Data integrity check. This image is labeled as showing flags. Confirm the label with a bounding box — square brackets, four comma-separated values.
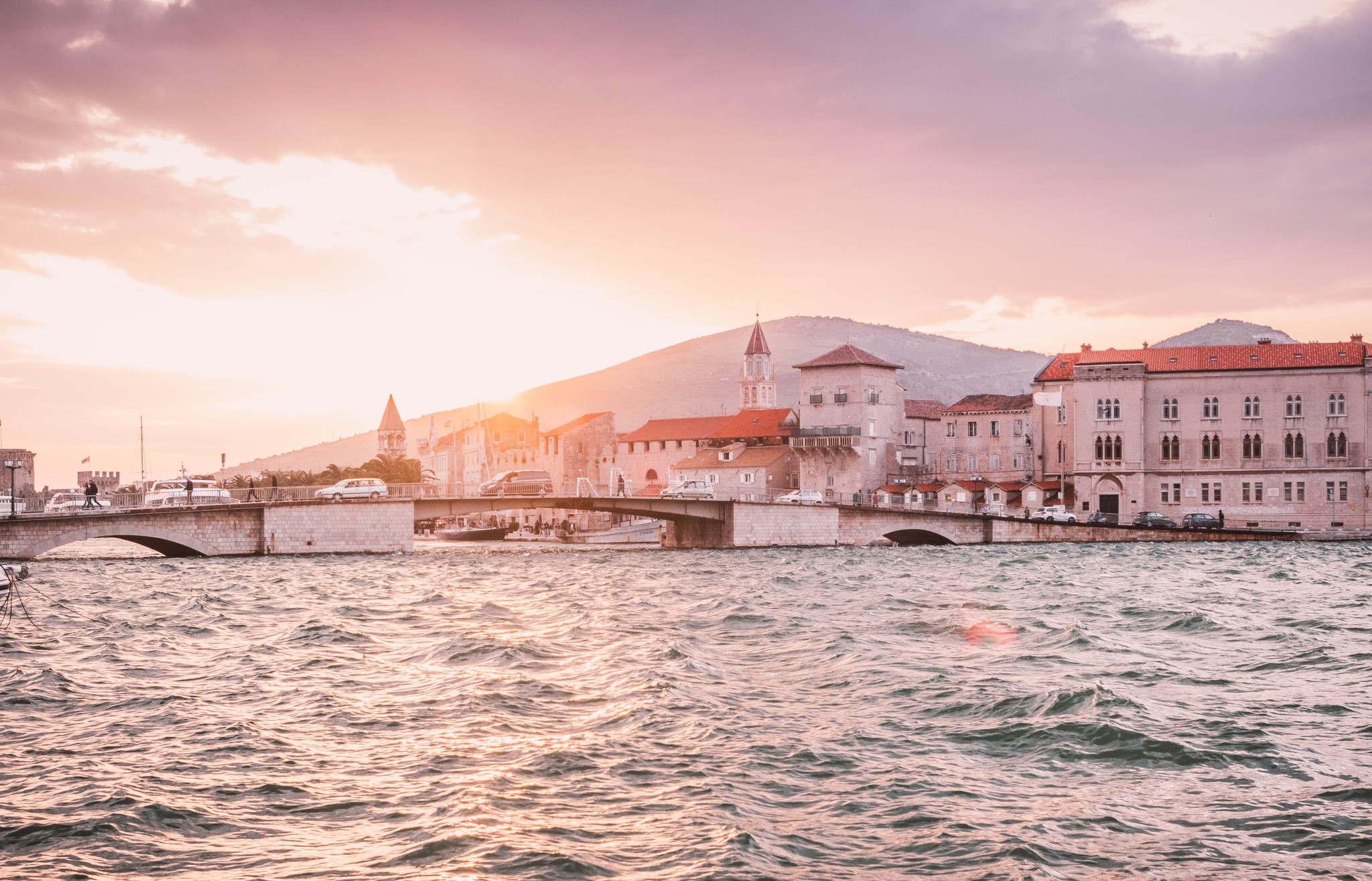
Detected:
[82, 456, 90, 464]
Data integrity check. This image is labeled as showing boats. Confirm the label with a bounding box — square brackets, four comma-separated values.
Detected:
[554, 512, 667, 543]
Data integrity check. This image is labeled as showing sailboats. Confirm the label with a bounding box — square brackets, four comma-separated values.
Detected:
[432, 401, 514, 542]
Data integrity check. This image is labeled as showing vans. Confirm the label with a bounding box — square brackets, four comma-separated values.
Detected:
[478, 470, 553, 498]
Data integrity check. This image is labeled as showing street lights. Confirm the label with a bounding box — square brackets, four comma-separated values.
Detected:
[3, 459, 23, 516]
[970, 474, 982, 513]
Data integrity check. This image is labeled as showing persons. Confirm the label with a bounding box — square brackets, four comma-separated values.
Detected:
[873, 492, 878, 507]
[267, 474, 278, 501]
[1024, 506, 1030, 518]
[570, 521, 574, 530]
[981, 505, 987, 514]
[82, 480, 103, 510]
[1218, 510, 1224, 523]
[853, 490, 864, 506]
[246, 478, 259, 502]
[1096, 509, 1102, 519]
[183, 478, 194, 505]
[539, 521, 549, 538]
[617, 475, 625, 496]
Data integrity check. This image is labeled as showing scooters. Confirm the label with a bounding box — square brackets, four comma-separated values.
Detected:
[1086, 513, 1107, 524]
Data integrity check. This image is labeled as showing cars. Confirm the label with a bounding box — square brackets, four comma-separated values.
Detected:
[1031, 507, 1078, 523]
[1182, 513, 1221, 529]
[314, 478, 389, 501]
[1132, 512, 1177, 528]
[660, 479, 714, 499]
[777, 489, 824, 504]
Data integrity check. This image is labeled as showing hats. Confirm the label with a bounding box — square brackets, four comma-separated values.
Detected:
[1219, 510, 1222, 511]
[618, 475, 622, 476]
[84, 481, 88, 484]
